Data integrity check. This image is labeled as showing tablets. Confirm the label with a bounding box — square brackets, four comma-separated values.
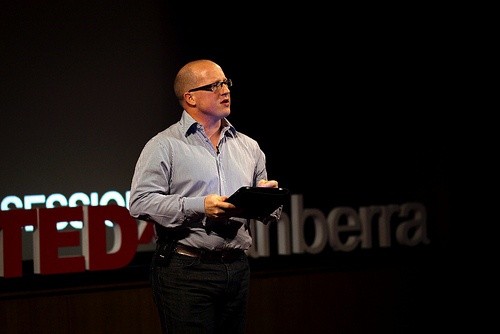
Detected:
[218, 186, 289, 220]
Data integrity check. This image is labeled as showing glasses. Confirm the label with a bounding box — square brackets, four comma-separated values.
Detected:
[188, 79, 232, 92]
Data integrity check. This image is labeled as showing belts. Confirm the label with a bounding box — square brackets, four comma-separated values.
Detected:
[159, 241, 248, 264]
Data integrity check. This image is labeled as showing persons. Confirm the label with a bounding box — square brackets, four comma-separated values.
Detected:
[130, 60, 283, 334]
